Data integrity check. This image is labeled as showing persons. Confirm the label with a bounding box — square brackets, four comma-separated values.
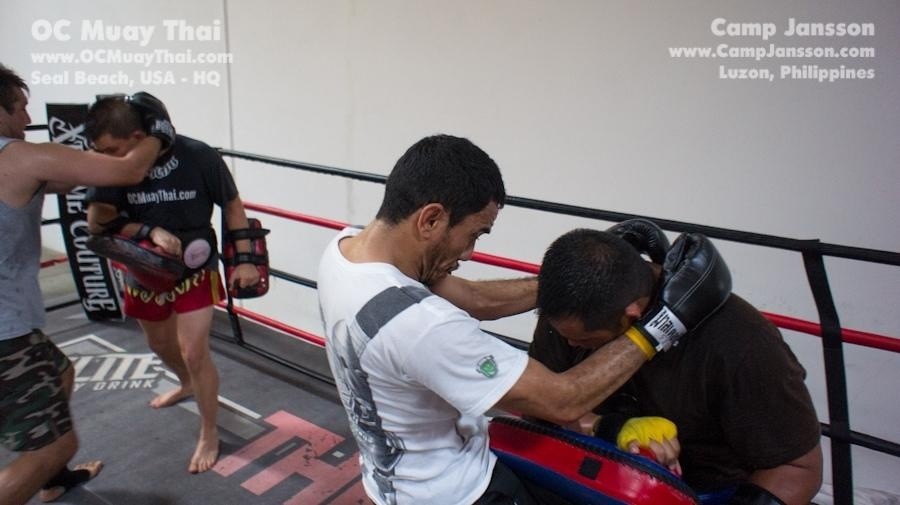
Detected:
[1, 60, 181, 505]
[525, 226, 825, 505]
[80, 92, 262, 477]
[314, 133, 734, 505]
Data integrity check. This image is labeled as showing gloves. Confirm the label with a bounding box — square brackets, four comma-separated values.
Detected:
[128, 90, 175, 157]
[604, 219, 671, 265]
[634, 229, 732, 353]
